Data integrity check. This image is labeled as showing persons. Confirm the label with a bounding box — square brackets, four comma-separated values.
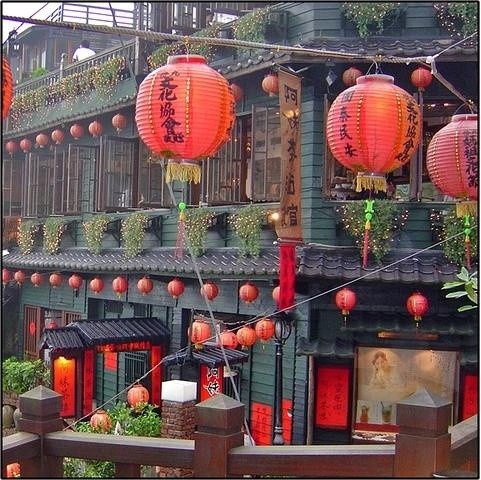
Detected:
[71, 41, 97, 63]
[370, 352, 402, 390]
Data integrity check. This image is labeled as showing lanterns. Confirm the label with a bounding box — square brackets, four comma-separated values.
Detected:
[3, 57, 14, 119]
[188, 320, 274, 351]
[343, 65, 363, 88]
[5, 112, 128, 158]
[408, 290, 428, 327]
[426, 113, 478, 272]
[201, 364, 223, 402]
[7, 463, 20, 477]
[134, 54, 236, 252]
[260, 73, 279, 97]
[1, 270, 280, 303]
[326, 73, 425, 268]
[91, 409, 110, 432]
[335, 287, 357, 323]
[127, 384, 149, 408]
[231, 83, 243, 104]
[411, 66, 433, 106]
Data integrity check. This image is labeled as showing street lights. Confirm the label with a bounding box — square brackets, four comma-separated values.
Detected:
[7, 27, 18, 81]
[267, 310, 295, 444]
